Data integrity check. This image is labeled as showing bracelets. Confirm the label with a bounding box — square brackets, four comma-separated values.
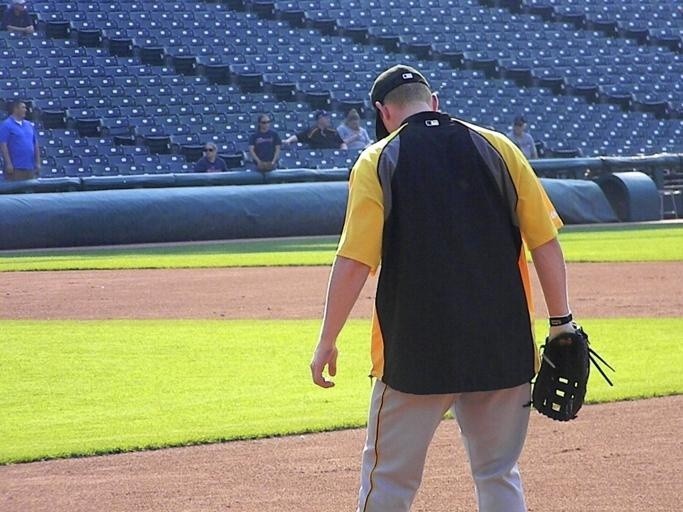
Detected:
[549, 313, 573, 326]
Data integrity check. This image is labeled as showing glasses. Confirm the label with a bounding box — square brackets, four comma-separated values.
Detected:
[259, 121, 270, 125]
[204, 149, 215, 152]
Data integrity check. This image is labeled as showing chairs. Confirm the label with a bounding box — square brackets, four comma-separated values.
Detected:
[0, 2, 683, 188]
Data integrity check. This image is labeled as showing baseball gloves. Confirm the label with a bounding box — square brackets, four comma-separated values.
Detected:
[533, 323, 589, 422]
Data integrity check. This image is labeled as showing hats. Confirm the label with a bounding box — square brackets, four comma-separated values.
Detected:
[370, 63, 432, 141]
[314, 108, 333, 120]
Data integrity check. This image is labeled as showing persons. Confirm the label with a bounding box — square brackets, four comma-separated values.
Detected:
[309, 65, 577, 512]
[192, 141, 229, 174]
[336, 109, 372, 149]
[281, 109, 349, 150]
[248, 113, 283, 171]
[2, 0, 35, 34]
[506, 116, 539, 161]
[0, 100, 42, 181]
